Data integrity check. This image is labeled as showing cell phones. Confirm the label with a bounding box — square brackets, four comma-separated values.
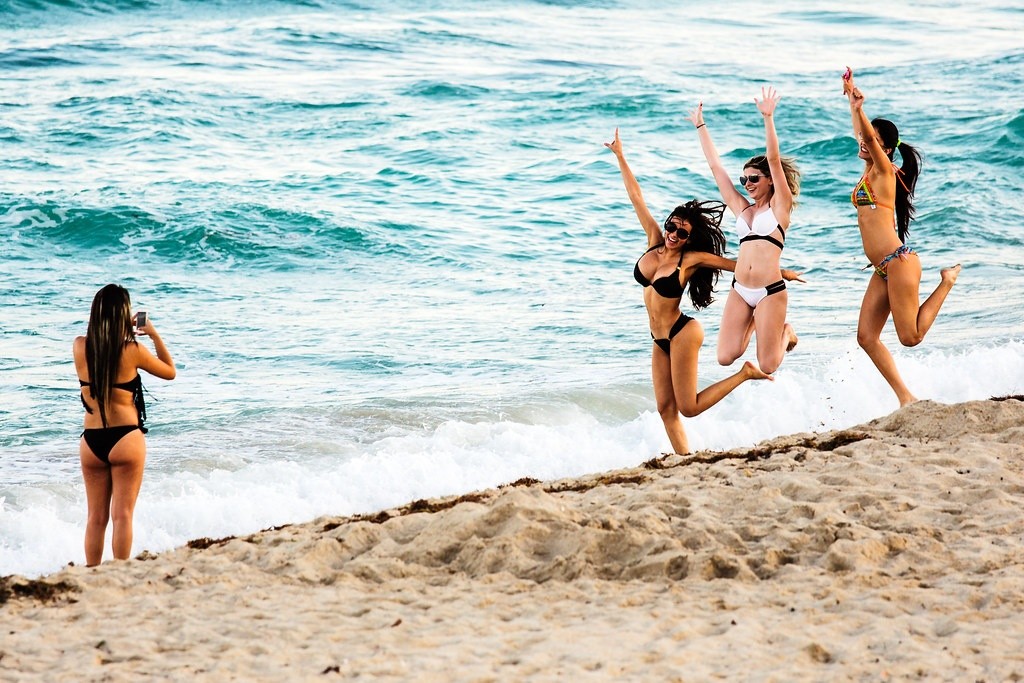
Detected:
[136, 311, 147, 330]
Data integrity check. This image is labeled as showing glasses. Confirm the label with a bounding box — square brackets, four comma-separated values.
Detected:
[740, 173, 767, 186]
[858, 129, 864, 141]
[664, 221, 690, 239]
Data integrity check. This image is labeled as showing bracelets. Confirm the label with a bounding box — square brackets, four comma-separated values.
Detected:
[697, 124, 705, 129]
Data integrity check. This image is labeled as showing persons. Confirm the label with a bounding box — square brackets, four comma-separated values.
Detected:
[603, 127, 807, 457]
[843, 66, 961, 408]
[684, 86, 801, 374]
[73, 284, 177, 566]
